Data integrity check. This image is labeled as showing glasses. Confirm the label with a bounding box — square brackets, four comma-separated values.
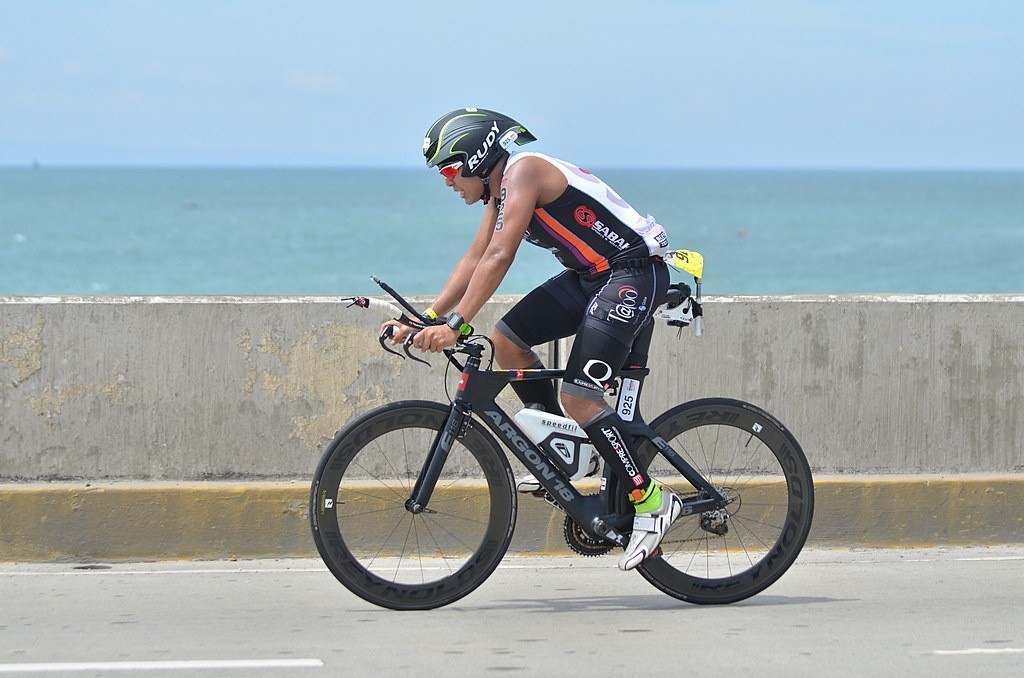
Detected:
[438, 160, 466, 179]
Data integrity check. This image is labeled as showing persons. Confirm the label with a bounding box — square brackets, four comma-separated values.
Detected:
[378, 109, 683, 571]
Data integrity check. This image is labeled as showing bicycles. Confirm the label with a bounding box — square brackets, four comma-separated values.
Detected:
[310, 275, 815, 612]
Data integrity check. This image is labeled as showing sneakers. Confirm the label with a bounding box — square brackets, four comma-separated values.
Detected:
[516, 450, 606, 493]
[617, 488, 683, 572]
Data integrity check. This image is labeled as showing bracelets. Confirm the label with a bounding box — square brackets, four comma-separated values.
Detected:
[421, 308, 439, 319]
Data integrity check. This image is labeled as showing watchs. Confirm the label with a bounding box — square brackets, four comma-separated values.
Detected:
[447, 311, 474, 338]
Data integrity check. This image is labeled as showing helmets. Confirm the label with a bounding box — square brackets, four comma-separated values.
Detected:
[424, 108, 535, 177]
